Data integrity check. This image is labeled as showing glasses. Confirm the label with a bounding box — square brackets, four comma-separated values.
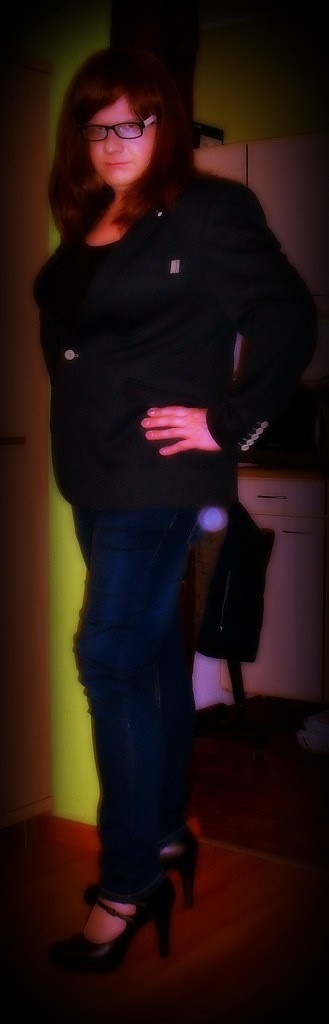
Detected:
[78, 114, 160, 142]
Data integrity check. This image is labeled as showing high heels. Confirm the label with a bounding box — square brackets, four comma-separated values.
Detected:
[83, 828, 199, 912]
[49, 869, 176, 975]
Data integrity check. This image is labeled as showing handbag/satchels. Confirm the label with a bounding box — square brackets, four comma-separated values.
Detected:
[171, 498, 275, 662]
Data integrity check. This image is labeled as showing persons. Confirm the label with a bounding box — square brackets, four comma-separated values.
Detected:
[31, 49, 317, 974]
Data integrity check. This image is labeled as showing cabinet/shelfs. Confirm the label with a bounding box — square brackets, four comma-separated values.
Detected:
[219, 478, 329, 703]
[192, 131, 329, 389]
